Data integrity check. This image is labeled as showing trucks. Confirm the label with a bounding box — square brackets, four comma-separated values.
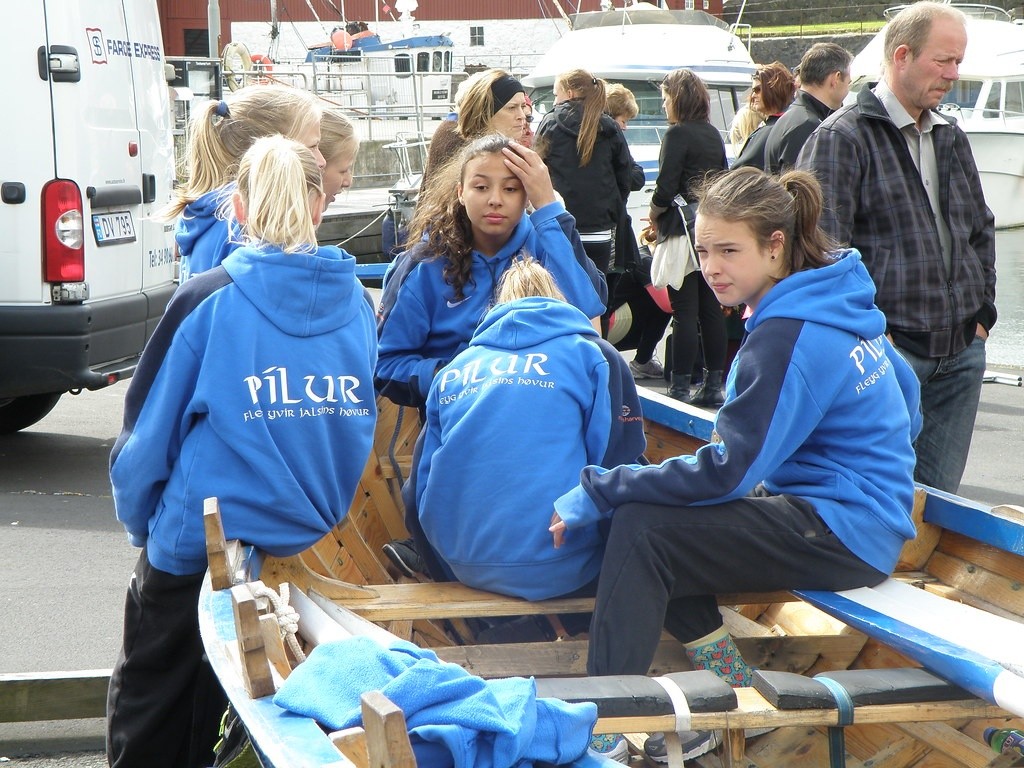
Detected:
[0, 0, 182, 431]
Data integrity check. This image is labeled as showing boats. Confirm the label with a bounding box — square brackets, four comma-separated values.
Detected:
[519, 0, 767, 246]
[162, 0, 471, 264]
[195, 382, 1024, 768]
[842, 1, 1024, 230]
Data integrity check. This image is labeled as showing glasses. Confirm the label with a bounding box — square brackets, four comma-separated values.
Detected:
[752, 85, 762, 93]
[526, 116, 534, 123]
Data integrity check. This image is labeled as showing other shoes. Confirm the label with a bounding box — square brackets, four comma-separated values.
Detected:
[476, 615, 591, 644]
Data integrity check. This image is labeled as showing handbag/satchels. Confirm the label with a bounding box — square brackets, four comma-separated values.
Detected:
[678, 202, 703, 269]
[611, 215, 647, 298]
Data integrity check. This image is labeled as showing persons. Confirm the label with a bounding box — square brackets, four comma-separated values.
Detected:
[795, 0, 998, 491]
[105, 134, 377, 768]
[168, 43, 857, 600]
[548, 166, 925, 762]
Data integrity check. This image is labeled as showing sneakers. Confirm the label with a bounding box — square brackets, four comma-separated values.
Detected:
[590, 739, 631, 766]
[382, 536, 431, 580]
[629, 359, 664, 379]
[644, 666, 779, 764]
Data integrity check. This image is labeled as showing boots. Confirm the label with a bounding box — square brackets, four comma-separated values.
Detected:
[668, 368, 724, 409]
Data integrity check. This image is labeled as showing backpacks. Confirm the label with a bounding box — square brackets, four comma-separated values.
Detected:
[665, 333, 704, 384]
